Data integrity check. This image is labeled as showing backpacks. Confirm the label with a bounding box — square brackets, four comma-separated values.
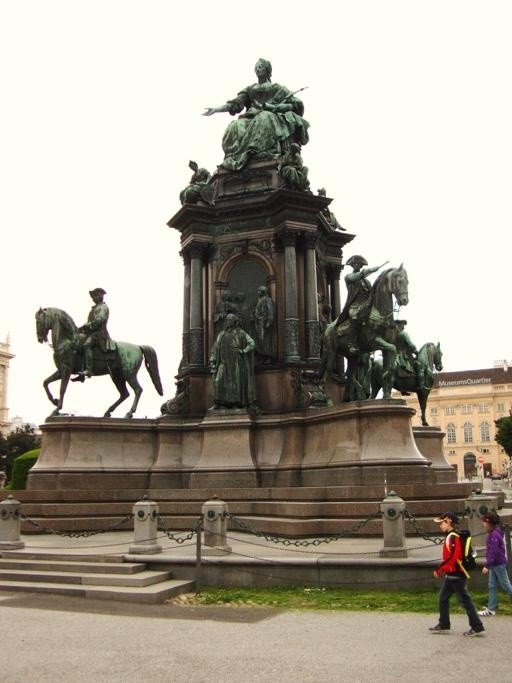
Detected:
[445, 529, 478, 573]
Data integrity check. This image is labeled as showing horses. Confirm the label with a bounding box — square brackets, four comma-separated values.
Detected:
[315, 261, 410, 403]
[34, 306, 165, 420]
[368, 340, 444, 427]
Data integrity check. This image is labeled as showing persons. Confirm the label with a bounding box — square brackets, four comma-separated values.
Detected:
[252, 285, 274, 364]
[179, 160, 212, 205]
[235, 288, 252, 320]
[200, 59, 311, 171]
[477, 511, 512, 617]
[278, 142, 309, 192]
[486, 470, 490, 477]
[394, 319, 417, 396]
[67, 288, 116, 382]
[213, 290, 239, 340]
[209, 313, 258, 411]
[0, 452, 9, 489]
[427, 511, 489, 637]
[339, 255, 380, 352]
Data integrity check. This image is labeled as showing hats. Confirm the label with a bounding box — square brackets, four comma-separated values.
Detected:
[433, 513, 458, 524]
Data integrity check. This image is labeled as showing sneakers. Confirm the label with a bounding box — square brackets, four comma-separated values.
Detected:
[428, 624, 451, 634]
[463, 627, 486, 636]
[477, 608, 496, 616]
